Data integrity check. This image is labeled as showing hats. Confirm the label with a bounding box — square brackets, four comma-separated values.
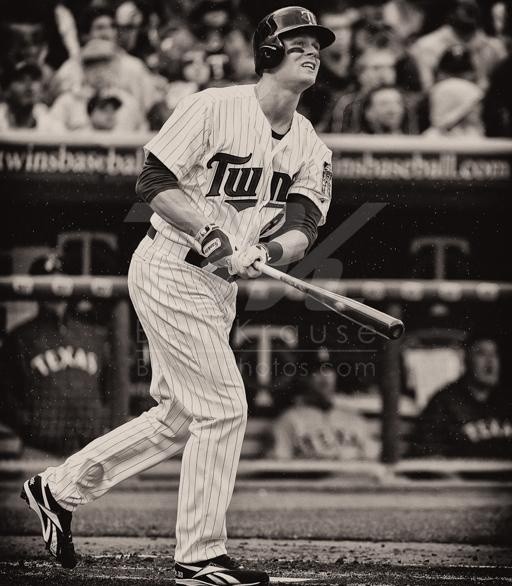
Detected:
[430, 78, 486, 128]
[16, 60, 41, 80]
[80, 39, 114, 59]
[87, 94, 121, 114]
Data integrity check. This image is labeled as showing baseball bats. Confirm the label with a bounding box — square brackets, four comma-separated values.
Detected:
[252, 260, 404, 342]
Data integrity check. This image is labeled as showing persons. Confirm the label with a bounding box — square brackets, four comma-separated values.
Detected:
[404, 329, 512, 483]
[268, 336, 383, 476]
[2, 246, 118, 457]
[18, 7, 333, 583]
[0, 1, 511, 138]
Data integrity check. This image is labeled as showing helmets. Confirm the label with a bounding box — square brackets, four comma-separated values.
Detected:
[252, 5, 337, 76]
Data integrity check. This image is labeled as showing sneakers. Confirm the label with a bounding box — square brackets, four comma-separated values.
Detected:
[21, 475, 82, 563]
[174, 554, 269, 586]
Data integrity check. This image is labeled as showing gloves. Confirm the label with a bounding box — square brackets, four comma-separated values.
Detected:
[229, 244, 270, 280]
[193, 221, 235, 267]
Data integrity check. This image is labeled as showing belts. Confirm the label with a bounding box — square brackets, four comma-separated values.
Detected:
[147, 227, 239, 284]
